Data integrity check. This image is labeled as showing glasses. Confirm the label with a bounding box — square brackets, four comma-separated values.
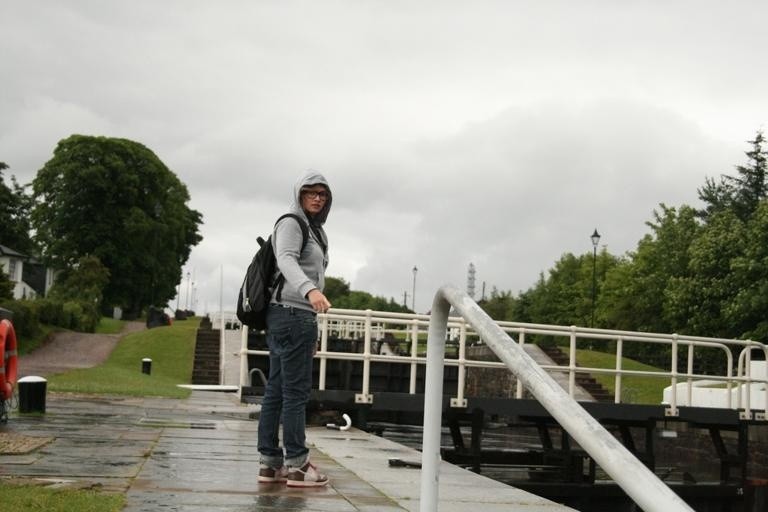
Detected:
[303, 189, 331, 202]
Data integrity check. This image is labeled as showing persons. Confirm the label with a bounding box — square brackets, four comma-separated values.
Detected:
[256, 168, 332, 488]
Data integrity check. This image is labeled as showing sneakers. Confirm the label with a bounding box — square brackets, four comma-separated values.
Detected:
[257, 461, 289, 483]
[285, 461, 330, 487]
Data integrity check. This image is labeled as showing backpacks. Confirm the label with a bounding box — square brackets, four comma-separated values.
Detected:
[234, 212, 311, 328]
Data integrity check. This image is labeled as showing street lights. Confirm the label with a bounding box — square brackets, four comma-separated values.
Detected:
[590, 228, 600, 351]
[412, 266, 418, 313]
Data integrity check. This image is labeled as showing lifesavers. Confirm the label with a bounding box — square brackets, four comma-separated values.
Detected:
[0, 319, 17, 400]
